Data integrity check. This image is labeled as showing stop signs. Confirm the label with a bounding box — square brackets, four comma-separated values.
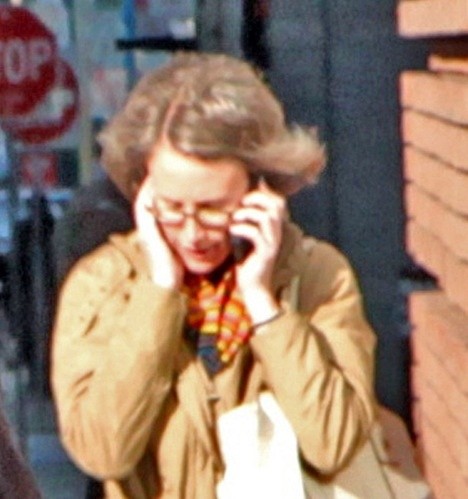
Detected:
[0, 5, 58, 113]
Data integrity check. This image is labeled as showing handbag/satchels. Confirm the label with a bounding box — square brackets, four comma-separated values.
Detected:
[216, 237, 429, 499]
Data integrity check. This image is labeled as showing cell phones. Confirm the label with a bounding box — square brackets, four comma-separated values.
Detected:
[230, 238, 254, 262]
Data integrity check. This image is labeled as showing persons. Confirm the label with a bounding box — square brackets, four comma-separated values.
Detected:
[50, 52, 433, 499]
[9, 186, 134, 397]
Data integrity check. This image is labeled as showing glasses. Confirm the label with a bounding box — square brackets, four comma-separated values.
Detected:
[146, 201, 241, 227]
